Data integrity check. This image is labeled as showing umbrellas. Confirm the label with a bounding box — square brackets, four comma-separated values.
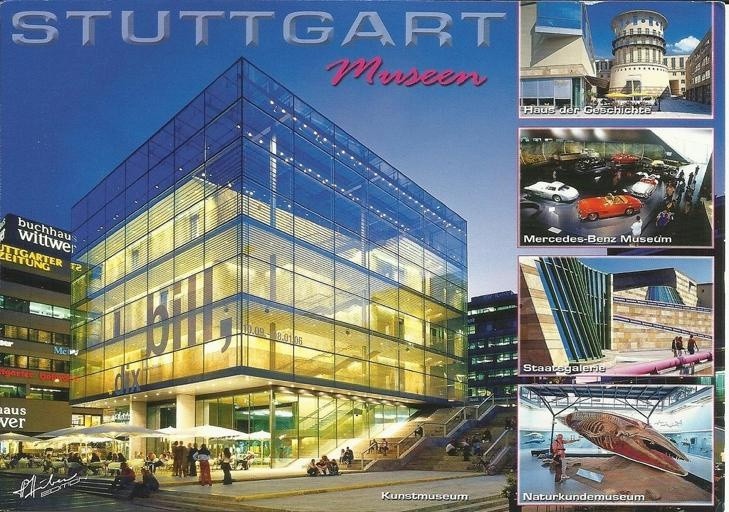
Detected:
[234, 429, 271, 460]
[0, 422, 249, 440]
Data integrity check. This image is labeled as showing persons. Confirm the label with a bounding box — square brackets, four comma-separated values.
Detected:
[687, 334, 699, 355]
[676, 336, 686, 358]
[306, 447, 354, 477]
[379, 438, 388, 456]
[444, 414, 517, 462]
[414, 425, 423, 437]
[109, 461, 135, 489]
[552, 450, 563, 484]
[672, 335, 679, 358]
[366, 438, 378, 454]
[144, 469, 160, 491]
[552, 434, 579, 478]
[127, 468, 148, 498]
[655, 164, 700, 229]
[629, 216, 644, 246]
[0, 440, 254, 486]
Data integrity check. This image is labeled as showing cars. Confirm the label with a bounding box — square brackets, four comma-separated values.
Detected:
[577, 156, 605, 171]
[577, 193, 644, 223]
[641, 157, 678, 176]
[523, 178, 579, 203]
[610, 153, 641, 165]
[630, 176, 659, 198]
